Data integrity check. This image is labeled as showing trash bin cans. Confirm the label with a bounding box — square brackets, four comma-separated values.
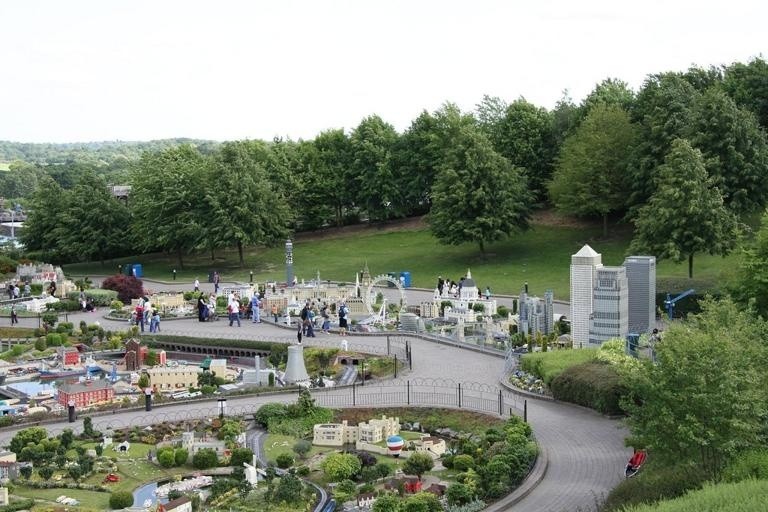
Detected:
[69, 404, 76, 422]
[145, 394, 151, 411]
[122, 263, 143, 278]
[387, 272, 396, 287]
[399, 272, 410, 288]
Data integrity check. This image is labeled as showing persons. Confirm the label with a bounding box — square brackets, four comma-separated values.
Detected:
[272, 304, 278, 322]
[438, 276, 481, 299]
[8, 279, 96, 323]
[486, 286, 491, 299]
[649, 328, 662, 363]
[297, 298, 350, 345]
[128, 296, 160, 332]
[195, 271, 261, 326]
